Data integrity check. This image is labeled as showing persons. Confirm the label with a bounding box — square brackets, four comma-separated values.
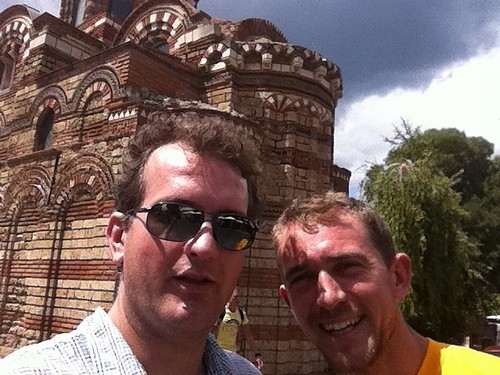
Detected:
[0, 113, 263, 375]
[271, 191, 500, 374]
[212, 287, 264, 370]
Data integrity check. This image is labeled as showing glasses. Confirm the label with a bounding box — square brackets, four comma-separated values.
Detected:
[125, 199, 260, 252]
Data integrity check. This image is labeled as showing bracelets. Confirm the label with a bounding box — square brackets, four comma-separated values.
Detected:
[255, 353, 261, 357]
